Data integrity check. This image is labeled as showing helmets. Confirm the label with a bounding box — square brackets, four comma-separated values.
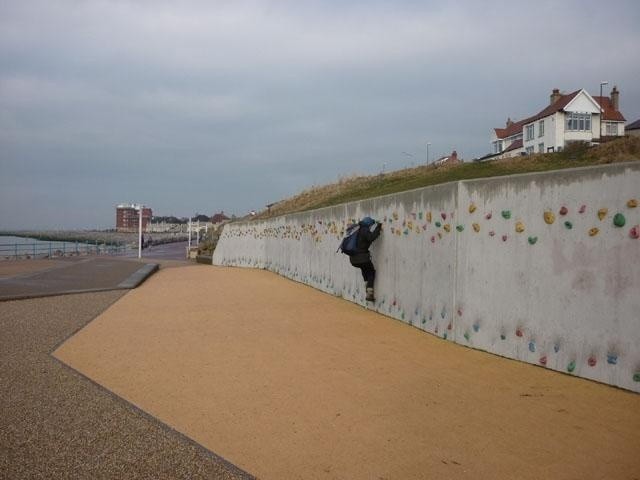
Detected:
[362, 217, 375, 227]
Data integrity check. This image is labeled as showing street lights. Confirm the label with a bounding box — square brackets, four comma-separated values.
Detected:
[597, 80, 609, 141]
[130, 202, 143, 261]
[425, 141, 432, 168]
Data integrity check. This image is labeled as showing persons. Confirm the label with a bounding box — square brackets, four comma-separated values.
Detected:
[350, 217, 382, 301]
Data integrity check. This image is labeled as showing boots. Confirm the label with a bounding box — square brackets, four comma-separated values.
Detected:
[364, 287, 377, 301]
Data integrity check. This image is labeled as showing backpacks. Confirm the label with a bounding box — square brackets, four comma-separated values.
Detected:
[340, 224, 372, 255]
